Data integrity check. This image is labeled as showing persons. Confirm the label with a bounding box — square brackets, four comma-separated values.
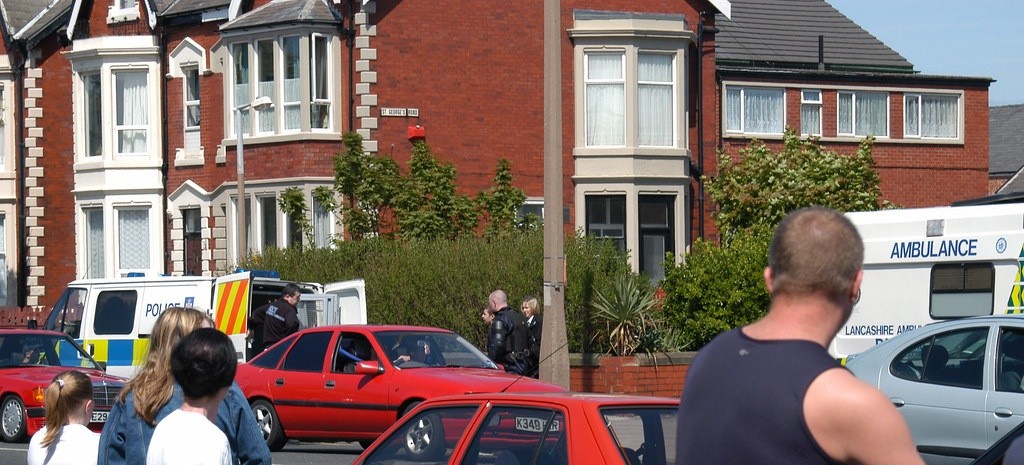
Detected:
[26, 370, 102, 465]
[97, 307, 273, 465]
[481, 306, 497, 353]
[519, 295, 543, 380]
[484, 290, 528, 376]
[145, 328, 238, 465]
[246, 283, 301, 357]
[673, 205, 927, 465]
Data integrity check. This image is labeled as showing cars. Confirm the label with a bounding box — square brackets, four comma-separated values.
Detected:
[231, 324, 579, 463]
[1, 325, 136, 445]
[351, 392, 683, 465]
[840, 312, 1024, 456]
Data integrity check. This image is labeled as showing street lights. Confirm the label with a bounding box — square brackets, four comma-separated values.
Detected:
[230, 96, 273, 272]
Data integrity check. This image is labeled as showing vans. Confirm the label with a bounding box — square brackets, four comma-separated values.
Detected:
[813, 191, 1024, 371]
[19, 263, 367, 413]
